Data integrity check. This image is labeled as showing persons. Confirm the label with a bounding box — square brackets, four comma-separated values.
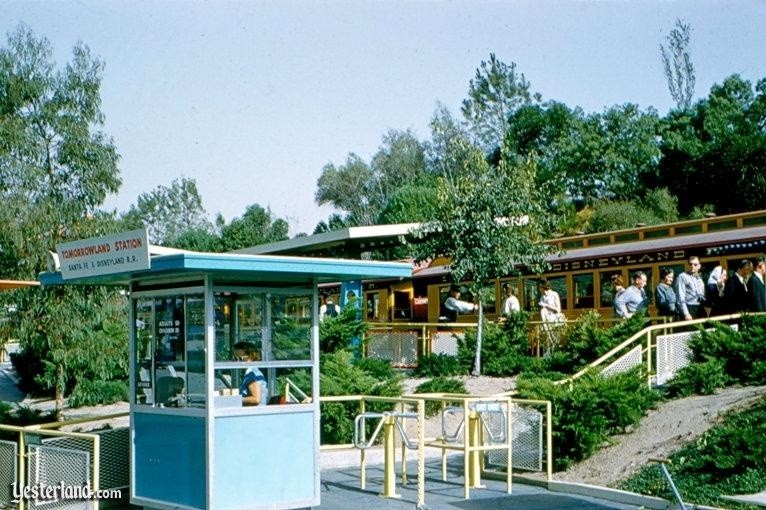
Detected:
[703, 265, 729, 316]
[743, 253, 766, 313]
[319, 295, 341, 323]
[536, 277, 562, 354]
[655, 266, 679, 324]
[230, 340, 267, 407]
[612, 271, 648, 318]
[501, 283, 520, 317]
[344, 291, 358, 312]
[610, 273, 626, 320]
[723, 257, 759, 313]
[673, 255, 709, 331]
[440, 284, 479, 322]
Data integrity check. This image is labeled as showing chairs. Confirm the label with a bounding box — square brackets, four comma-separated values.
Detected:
[267, 396, 286, 405]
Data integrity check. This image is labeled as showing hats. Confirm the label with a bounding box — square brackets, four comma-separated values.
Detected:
[450, 284, 461, 292]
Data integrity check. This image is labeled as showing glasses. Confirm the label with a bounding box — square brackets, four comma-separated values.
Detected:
[689, 263, 697, 266]
[233, 355, 248, 361]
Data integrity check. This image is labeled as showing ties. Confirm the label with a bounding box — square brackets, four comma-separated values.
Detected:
[743, 278, 748, 292]
[638, 287, 648, 307]
